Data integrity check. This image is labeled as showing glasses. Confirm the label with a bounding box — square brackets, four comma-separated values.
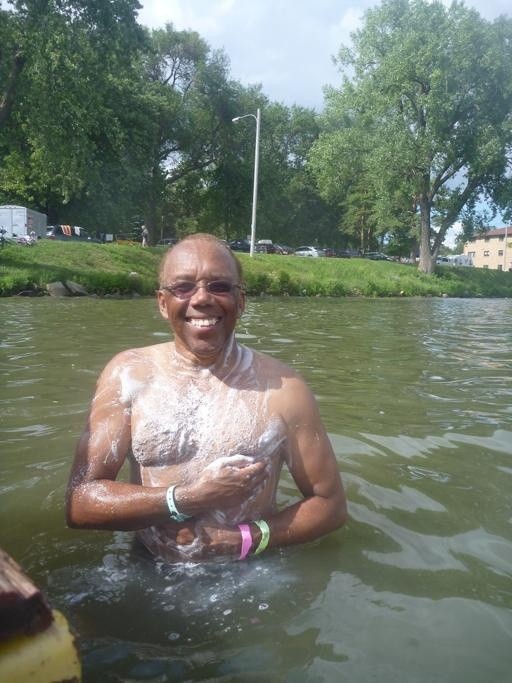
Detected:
[159, 281, 242, 300]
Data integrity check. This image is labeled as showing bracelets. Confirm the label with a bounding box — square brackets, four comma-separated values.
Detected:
[251, 514, 271, 556]
[165, 484, 193, 524]
[237, 521, 253, 562]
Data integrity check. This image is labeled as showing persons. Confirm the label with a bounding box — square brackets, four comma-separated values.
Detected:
[140, 223, 149, 247]
[63, 231, 349, 569]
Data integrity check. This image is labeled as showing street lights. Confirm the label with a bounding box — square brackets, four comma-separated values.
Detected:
[502, 220, 511, 270]
[231, 106, 262, 256]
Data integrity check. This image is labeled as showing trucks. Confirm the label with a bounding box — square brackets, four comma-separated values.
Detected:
[1, 204, 48, 242]
[436, 253, 474, 267]
